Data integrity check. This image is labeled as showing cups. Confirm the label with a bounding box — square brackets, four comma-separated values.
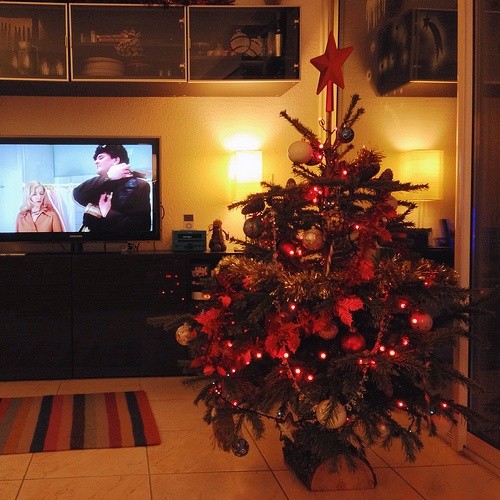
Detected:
[207, 50, 230, 57]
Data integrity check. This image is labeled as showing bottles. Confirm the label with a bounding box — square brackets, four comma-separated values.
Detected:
[273, 29, 282, 56]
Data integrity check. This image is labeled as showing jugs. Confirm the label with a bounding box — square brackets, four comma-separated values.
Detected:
[16, 41, 39, 75]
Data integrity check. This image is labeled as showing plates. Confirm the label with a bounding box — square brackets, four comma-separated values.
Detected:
[79, 57, 153, 77]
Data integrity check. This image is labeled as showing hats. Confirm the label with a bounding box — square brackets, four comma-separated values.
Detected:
[93, 144, 129, 164]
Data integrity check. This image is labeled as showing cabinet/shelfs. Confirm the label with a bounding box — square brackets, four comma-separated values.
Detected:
[376, 7, 457, 96]
[0, 251, 246, 382]
[0, 1, 302, 98]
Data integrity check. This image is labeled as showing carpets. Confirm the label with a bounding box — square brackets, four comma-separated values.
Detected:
[0, 391, 161, 454]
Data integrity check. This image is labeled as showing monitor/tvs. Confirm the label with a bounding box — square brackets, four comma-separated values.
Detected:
[0, 137, 161, 256]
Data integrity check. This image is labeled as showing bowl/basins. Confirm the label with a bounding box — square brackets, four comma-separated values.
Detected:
[191, 42, 209, 56]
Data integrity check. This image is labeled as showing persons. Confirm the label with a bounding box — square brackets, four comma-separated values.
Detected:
[16, 179, 62, 232]
[73, 144, 151, 233]
